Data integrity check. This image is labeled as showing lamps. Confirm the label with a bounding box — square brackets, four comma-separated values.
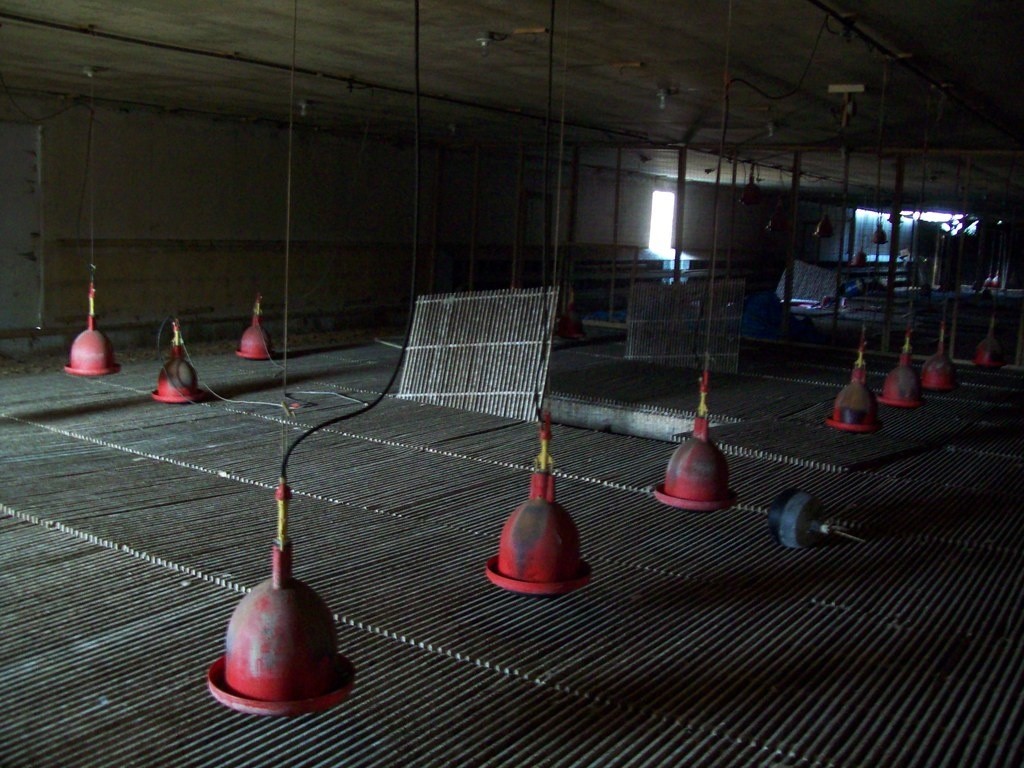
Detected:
[59, 0, 1007, 718]
